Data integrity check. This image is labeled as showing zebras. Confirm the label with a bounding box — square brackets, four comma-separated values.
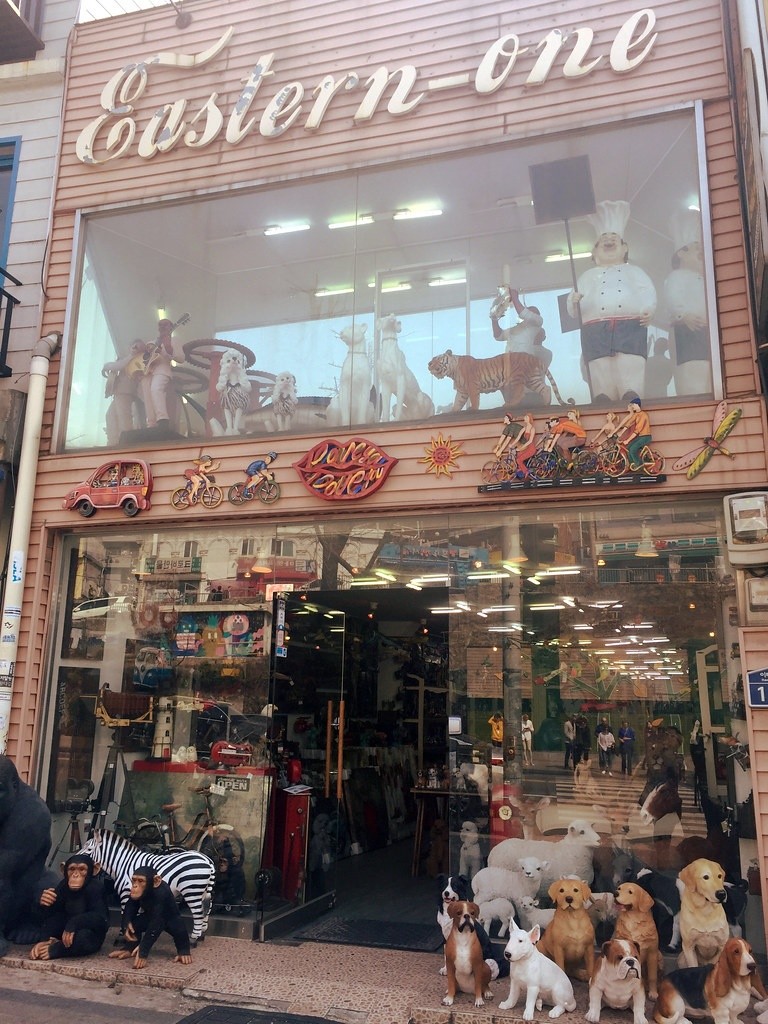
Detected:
[76, 827, 216, 948]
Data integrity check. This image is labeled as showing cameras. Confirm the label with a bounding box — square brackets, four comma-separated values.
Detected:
[60, 798, 88, 815]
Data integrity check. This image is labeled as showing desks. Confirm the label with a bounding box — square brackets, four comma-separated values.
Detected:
[409, 787, 486, 879]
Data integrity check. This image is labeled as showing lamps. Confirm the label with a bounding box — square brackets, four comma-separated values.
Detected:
[252, 547, 273, 574]
[390, 205, 446, 223]
[325, 216, 376, 230]
[256, 223, 313, 238]
[502, 522, 531, 564]
[634, 515, 664, 559]
[309, 283, 355, 300]
[536, 248, 595, 270]
[368, 282, 412, 297]
[428, 278, 468, 289]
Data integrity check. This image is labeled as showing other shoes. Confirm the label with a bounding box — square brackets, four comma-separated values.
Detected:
[628, 772, 632, 776]
[609, 772, 612, 776]
[531, 762, 535, 765]
[602, 771, 605, 774]
[525, 762, 529, 765]
[623, 769, 625, 774]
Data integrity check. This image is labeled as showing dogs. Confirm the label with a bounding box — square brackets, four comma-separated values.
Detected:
[499, 917, 576, 1020]
[377, 314, 434, 420]
[216, 349, 252, 435]
[678, 857, 732, 969]
[434, 821, 510, 981]
[326, 322, 375, 427]
[535, 878, 595, 980]
[609, 881, 664, 1001]
[272, 372, 299, 432]
[582, 936, 757, 1024]
[440, 901, 493, 1007]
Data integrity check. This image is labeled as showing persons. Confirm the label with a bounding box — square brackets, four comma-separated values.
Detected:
[102, 339, 145, 444]
[488, 711, 504, 747]
[567, 200, 657, 402]
[142, 319, 184, 429]
[490, 286, 543, 354]
[641, 721, 656, 770]
[665, 209, 712, 396]
[598, 726, 616, 777]
[638, 727, 685, 869]
[88, 585, 109, 598]
[618, 720, 635, 777]
[521, 714, 535, 766]
[595, 717, 613, 770]
[564, 714, 590, 770]
[207, 585, 232, 602]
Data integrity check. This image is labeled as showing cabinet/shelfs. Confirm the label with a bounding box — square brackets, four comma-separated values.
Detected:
[404, 672, 449, 768]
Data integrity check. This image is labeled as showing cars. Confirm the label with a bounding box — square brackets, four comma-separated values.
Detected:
[70, 596, 135, 622]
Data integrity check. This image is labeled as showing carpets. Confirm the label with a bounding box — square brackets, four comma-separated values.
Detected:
[292, 914, 443, 954]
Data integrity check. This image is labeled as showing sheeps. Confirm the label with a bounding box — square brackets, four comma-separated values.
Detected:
[486, 819, 602, 898]
[519, 895, 557, 929]
[470, 857, 550, 938]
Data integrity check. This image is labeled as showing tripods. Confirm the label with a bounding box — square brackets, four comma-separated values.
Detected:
[48, 811, 82, 868]
[87, 727, 141, 853]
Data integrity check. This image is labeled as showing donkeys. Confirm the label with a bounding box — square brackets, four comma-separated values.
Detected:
[610, 840, 749, 953]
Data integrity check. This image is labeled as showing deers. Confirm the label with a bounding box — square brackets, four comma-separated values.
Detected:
[509, 793, 635, 855]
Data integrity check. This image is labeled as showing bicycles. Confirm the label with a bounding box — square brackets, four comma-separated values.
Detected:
[125, 786, 245, 875]
